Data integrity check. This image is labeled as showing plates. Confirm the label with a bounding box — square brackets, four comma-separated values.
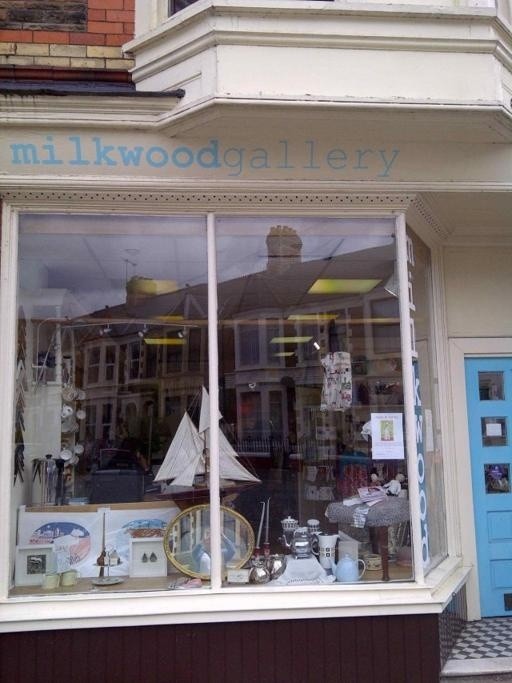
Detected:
[94, 578, 124, 584]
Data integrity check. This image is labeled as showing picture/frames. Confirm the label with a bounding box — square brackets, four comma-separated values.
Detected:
[12, 543, 58, 589]
[129, 537, 168, 578]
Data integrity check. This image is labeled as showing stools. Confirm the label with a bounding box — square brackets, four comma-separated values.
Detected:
[326, 495, 414, 583]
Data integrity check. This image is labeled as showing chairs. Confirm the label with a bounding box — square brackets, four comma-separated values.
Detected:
[91, 468, 144, 503]
[97, 447, 139, 470]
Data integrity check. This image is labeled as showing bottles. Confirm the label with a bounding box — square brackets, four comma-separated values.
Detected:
[306, 518, 322, 538]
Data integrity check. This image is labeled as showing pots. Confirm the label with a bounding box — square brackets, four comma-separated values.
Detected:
[30, 452, 66, 506]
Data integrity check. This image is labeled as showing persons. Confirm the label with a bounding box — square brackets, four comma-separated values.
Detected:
[369, 472, 380, 485]
[191, 528, 237, 575]
[382, 472, 406, 497]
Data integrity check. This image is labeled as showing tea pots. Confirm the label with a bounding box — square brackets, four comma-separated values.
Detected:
[327, 554, 365, 582]
[311, 531, 340, 569]
[280, 516, 298, 553]
[248, 555, 271, 583]
[280, 530, 317, 559]
[266, 553, 286, 580]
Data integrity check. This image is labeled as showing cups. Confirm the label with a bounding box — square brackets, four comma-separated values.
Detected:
[42, 573, 61, 590]
[363, 553, 382, 570]
[61, 569, 82, 587]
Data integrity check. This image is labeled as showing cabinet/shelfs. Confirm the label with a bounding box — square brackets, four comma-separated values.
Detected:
[23, 381, 75, 505]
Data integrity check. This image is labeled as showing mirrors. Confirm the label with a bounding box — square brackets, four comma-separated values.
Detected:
[163, 501, 256, 580]
